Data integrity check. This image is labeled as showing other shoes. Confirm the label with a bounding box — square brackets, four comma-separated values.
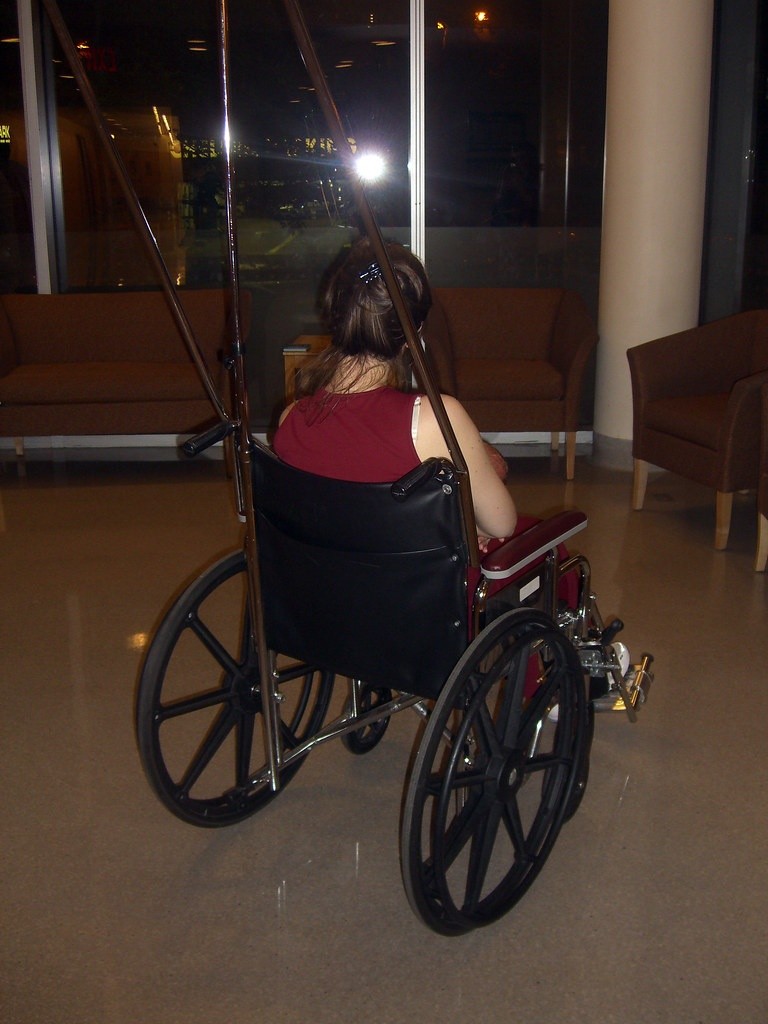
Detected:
[549, 642, 630, 721]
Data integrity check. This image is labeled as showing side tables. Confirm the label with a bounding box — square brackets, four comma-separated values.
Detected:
[283, 335, 332, 406]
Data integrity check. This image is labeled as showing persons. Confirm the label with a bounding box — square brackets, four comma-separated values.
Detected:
[275, 239, 631, 722]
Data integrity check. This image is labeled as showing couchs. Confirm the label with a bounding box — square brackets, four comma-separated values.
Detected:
[625, 307, 768, 551]
[425, 286, 599, 481]
[0, 289, 251, 479]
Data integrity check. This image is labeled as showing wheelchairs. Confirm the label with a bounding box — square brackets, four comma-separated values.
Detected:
[50, 0, 653, 939]
